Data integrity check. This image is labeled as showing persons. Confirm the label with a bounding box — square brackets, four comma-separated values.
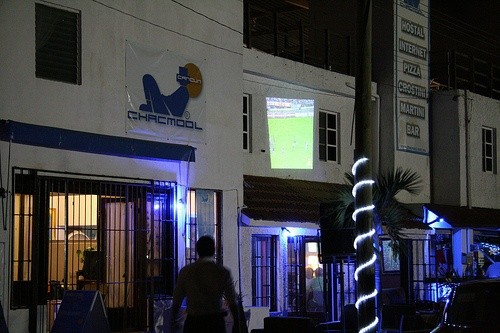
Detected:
[470, 252, 485, 276]
[305, 267, 329, 312]
[172, 236, 249, 333]
[486, 255, 500, 278]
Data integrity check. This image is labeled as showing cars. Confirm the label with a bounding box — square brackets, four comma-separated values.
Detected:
[429, 278, 500, 333]
